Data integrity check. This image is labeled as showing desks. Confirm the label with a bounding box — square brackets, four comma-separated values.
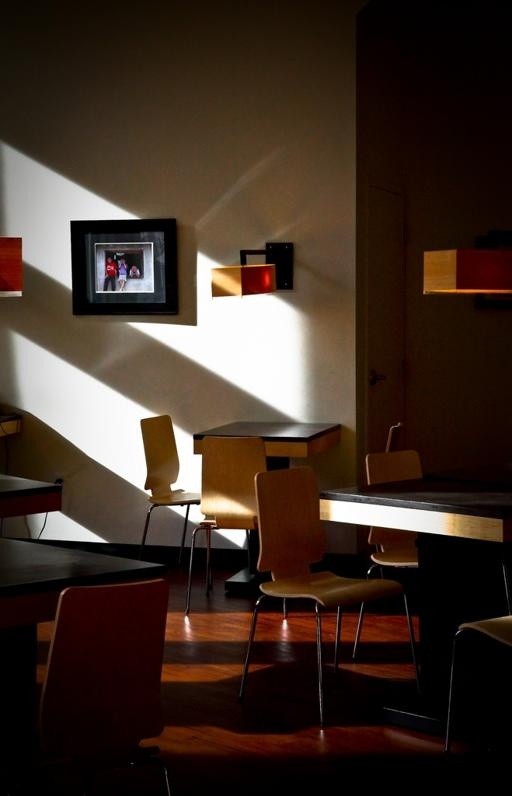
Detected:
[1, 532, 169, 612]
[191, 421, 343, 603]
[317, 474, 510, 546]
[0, 411, 23, 439]
[0, 471, 63, 519]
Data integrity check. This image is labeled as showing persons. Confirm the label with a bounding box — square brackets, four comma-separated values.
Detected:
[103, 256, 118, 290]
[117, 257, 128, 291]
[129, 264, 141, 278]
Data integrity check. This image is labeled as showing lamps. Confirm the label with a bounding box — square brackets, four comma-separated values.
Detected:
[209, 239, 294, 297]
[421, 247, 512, 301]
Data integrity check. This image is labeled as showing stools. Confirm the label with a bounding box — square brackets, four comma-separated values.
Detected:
[442, 611, 511, 757]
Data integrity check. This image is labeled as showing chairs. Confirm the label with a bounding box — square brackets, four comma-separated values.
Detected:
[351, 448, 423, 662]
[138, 415, 215, 592]
[185, 434, 269, 617]
[36, 578, 174, 796]
[237, 463, 424, 732]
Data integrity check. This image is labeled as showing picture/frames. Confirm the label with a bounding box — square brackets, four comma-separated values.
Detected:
[69, 216, 181, 317]
